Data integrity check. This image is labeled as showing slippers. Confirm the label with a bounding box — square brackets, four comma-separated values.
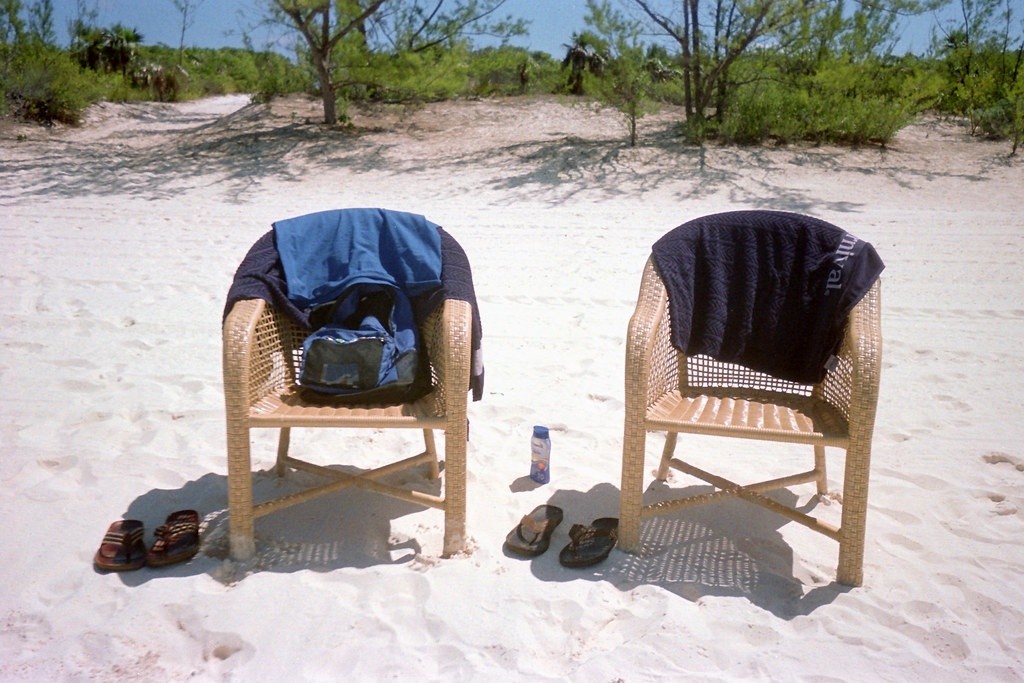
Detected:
[560, 517, 619, 566]
[94, 520, 147, 571]
[149, 510, 200, 566]
[506, 505, 563, 554]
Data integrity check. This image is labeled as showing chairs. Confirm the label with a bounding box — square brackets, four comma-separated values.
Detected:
[221, 205, 485, 556]
[617, 210, 887, 589]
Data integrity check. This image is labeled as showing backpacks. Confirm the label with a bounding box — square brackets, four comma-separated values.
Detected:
[299, 284, 433, 402]
